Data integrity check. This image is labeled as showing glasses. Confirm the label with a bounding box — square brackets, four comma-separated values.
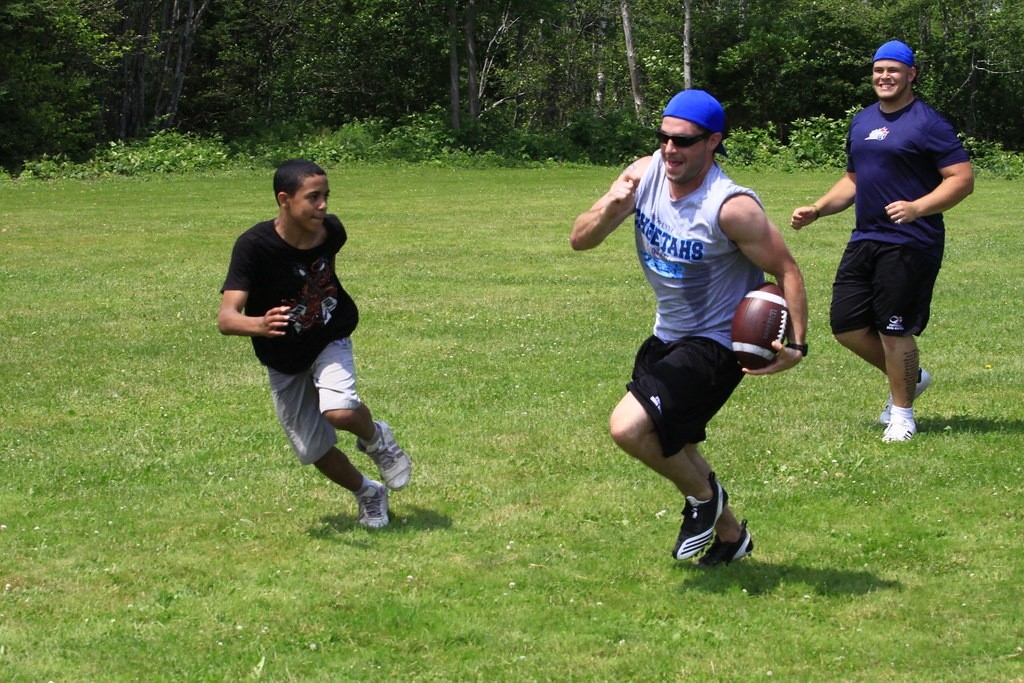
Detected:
[657, 131, 711, 147]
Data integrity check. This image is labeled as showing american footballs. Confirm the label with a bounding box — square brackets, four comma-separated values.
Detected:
[731, 281, 790, 370]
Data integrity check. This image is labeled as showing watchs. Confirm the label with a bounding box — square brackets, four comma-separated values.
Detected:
[785, 342, 809, 357]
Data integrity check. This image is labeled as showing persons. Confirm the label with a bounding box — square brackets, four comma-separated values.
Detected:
[216, 156, 411, 530]
[569, 88, 810, 563]
[790, 41, 976, 443]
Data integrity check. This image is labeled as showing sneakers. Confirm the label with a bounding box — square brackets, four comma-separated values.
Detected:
[880, 419, 915, 444]
[673, 470, 730, 560]
[700, 519, 755, 567]
[355, 482, 389, 528]
[357, 421, 413, 491]
[880, 366, 932, 423]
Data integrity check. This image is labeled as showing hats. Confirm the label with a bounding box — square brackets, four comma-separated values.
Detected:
[874, 39, 915, 67]
[663, 90, 728, 159]
